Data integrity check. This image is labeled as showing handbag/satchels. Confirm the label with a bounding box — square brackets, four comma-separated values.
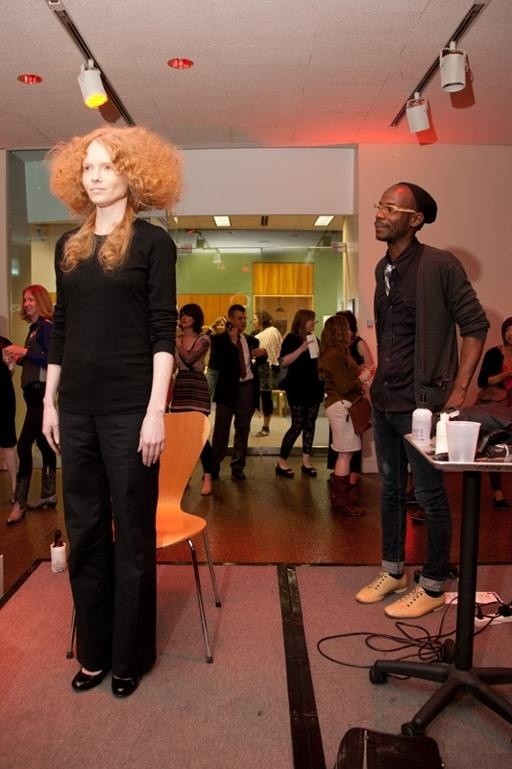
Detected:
[347, 394, 373, 436]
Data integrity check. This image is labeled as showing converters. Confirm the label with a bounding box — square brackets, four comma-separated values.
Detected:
[497, 606, 512, 616]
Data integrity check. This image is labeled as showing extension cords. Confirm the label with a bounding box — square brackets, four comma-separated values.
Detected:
[475, 612, 511, 626]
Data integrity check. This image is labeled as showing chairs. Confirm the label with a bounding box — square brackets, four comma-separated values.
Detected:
[64, 406, 226, 665]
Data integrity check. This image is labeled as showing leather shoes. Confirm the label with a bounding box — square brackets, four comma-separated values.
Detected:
[355, 573, 410, 604]
[71, 668, 106, 692]
[111, 673, 140, 698]
[494, 500, 509, 511]
[7, 502, 25, 523]
[383, 585, 446, 619]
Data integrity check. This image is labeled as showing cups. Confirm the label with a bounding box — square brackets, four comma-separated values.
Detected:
[4, 352, 16, 371]
[445, 421, 482, 463]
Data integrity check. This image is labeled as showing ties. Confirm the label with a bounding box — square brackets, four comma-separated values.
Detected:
[237, 334, 247, 380]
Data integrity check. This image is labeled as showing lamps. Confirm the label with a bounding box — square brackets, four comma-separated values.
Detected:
[401, 37, 473, 137]
[188, 228, 206, 249]
[315, 228, 334, 249]
[75, 52, 108, 110]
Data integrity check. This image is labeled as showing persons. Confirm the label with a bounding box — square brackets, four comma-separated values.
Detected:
[354, 182, 492, 619]
[1, 282, 376, 527]
[41, 124, 186, 698]
[475, 316, 512, 512]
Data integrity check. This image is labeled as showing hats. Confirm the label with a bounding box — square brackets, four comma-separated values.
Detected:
[399, 182, 437, 224]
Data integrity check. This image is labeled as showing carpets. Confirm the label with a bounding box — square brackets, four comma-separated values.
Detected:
[290, 562, 512, 768]
[2, 558, 311, 768]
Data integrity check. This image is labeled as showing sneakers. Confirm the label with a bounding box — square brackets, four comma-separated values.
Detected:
[410, 508, 426, 522]
[406, 489, 418, 504]
[200, 471, 220, 495]
[232, 470, 246, 480]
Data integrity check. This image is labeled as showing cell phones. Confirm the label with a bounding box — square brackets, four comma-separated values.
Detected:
[225, 321, 234, 331]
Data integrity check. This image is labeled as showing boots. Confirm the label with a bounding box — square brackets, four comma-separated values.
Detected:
[330, 473, 366, 517]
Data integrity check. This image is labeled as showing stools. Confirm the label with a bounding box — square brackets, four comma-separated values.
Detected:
[271, 389, 290, 417]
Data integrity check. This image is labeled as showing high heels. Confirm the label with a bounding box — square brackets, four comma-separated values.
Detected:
[29, 496, 57, 511]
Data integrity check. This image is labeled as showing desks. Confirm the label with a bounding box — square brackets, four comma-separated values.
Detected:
[366, 428, 512, 743]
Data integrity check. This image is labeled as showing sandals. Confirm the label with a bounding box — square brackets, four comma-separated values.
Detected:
[301, 464, 317, 477]
[275, 462, 295, 477]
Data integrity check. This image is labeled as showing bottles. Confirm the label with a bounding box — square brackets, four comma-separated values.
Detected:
[436, 410, 460, 456]
[49, 529, 69, 573]
[411, 394, 432, 448]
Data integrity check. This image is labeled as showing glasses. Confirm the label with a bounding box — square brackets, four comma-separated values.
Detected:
[372, 204, 417, 215]
